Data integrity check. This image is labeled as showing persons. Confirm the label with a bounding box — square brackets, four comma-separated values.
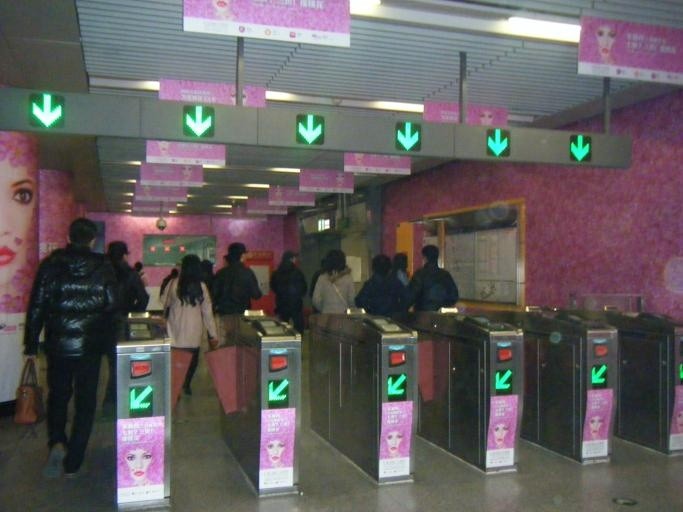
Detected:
[308, 259, 325, 299]
[311, 249, 356, 313]
[596, 26, 618, 62]
[355, 254, 409, 317]
[160, 254, 219, 395]
[124, 448, 153, 483]
[213, 243, 262, 344]
[492, 423, 509, 447]
[22, 217, 116, 483]
[391, 254, 411, 287]
[266, 439, 286, 468]
[1, 160, 38, 300]
[98, 240, 149, 402]
[588, 416, 604, 438]
[676, 411, 683, 433]
[385, 431, 404, 458]
[391, 244, 459, 314]
[213, 0, 237, 20]
[269, 251, 307, 339]
[134, 261, 145, 277]
[200, 260, 217, 311]
[159, 269, 178, 320]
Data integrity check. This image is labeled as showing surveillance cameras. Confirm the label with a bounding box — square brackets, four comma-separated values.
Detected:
[156, 220, 167, 230]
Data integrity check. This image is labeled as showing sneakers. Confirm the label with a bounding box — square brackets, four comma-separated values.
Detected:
[63, 469, 81, 479]
[40, 441, 67, 481]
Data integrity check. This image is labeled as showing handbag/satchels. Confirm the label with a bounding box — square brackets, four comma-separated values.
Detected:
[13, 358, 47, 426]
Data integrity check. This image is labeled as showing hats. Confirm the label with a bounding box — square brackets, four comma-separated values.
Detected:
[282, 250, 300, 260]
